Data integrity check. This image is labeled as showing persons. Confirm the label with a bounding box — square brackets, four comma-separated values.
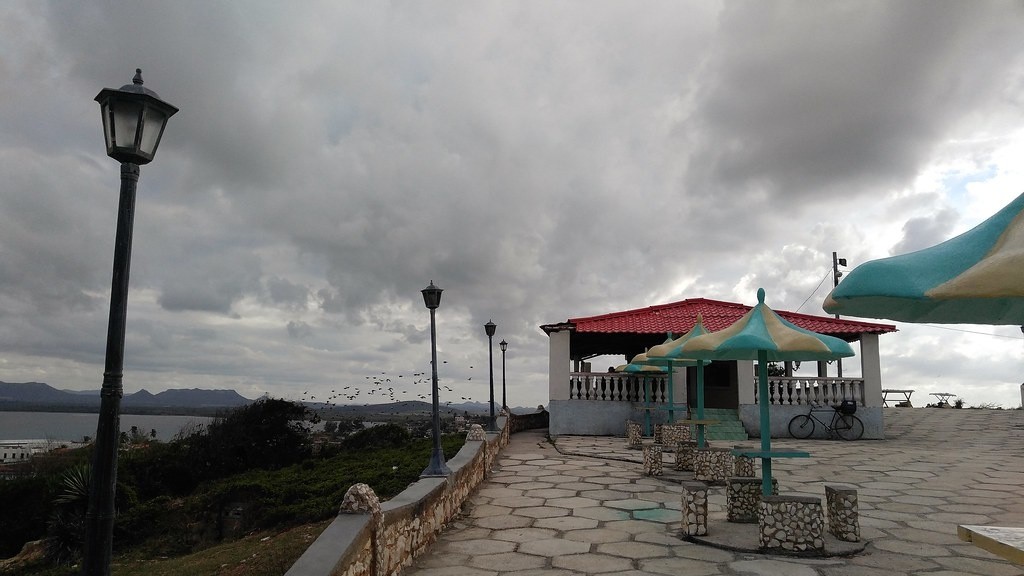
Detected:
[601, 367, 622, 395]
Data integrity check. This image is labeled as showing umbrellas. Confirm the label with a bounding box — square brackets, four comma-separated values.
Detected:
[646, 314, 711, 448]
[682, 288, 856, 496]
[615, 348, 678, 436]
[823, 193, 1024, 324]
[631, 332, 712, 423]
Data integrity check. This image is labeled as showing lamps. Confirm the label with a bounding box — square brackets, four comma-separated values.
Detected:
[792, 360, 801, 372]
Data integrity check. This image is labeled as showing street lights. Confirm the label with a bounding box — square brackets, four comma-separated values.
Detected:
[80, 70, 182, 576]
[484, 318, 501, 434]
[499, 338, 508, 413]
[419, 278, 453, 477]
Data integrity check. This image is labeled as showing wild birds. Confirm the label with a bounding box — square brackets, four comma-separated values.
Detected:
[291, 360, 488, 436]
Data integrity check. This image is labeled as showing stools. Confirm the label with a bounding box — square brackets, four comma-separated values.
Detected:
[725, 477, 779, 524]
[642, 443, 663, 475]
[733, 444, 756, 478]
[654, 424, 707, 447]
[758, 494, 823, 553]
[625, 418, 642, 450]
[675, 440, 708, 472]
[682, 480, 709, 537]
[824, 484, 860, 542]
[692, 447, 733, 484]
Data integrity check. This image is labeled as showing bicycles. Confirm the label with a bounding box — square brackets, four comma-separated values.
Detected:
[789, 399, 864, 441]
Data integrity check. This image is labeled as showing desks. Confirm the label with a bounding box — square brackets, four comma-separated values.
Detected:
[732, 447, 811, 494]
[678, 419, 720, 446]
[882, 389, 914, 408]
[929, 393, 956, 408]
[637, 406, 656, 437]
[658, 406, 688, 425]
